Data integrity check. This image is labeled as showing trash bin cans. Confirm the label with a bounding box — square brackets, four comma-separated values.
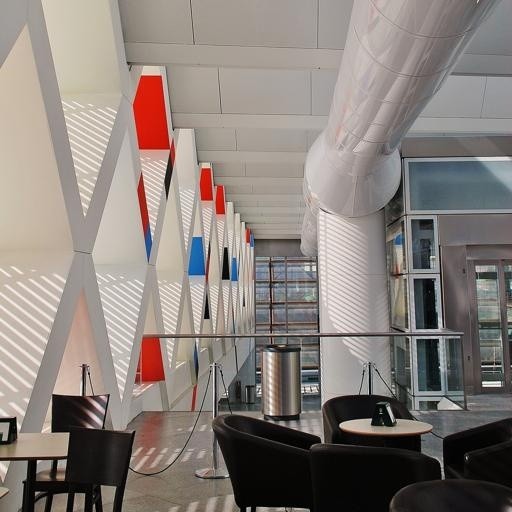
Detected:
[261, 343, 301, 421]
[245, 385, 255, 404]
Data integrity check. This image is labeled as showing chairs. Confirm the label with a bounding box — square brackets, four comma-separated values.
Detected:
[24, 395, 136, 511]
[322, 396, 425, 450]
[306, 442, 442, 511]
[389, 478, 512, 511]
[441, 416, 511, 480]
[212, 412, 325, 510]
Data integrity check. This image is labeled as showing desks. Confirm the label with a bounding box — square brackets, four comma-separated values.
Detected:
[0, 431, 71, 510]
[338, 416, 436, 439]
